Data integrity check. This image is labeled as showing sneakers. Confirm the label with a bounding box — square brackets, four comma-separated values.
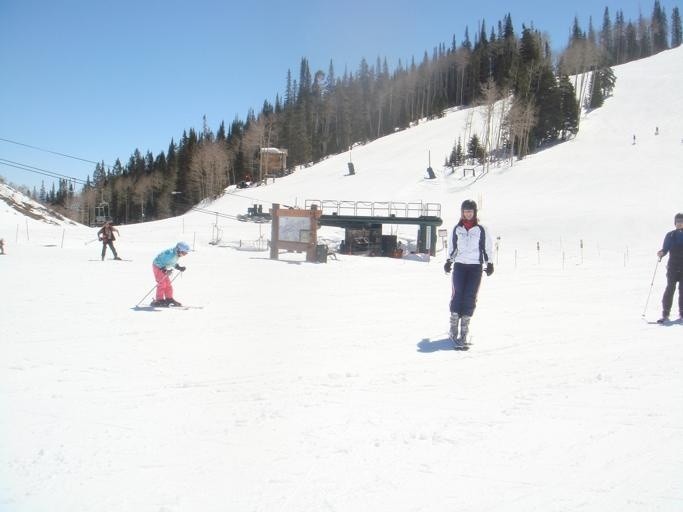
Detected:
[657, 317, 670, 323]
[155, 298, 181, 306]
[450, 333, 467, 344]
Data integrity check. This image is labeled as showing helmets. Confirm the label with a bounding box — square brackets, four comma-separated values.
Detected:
[176, 241, 190, 252]
[462, 199, 478, 211]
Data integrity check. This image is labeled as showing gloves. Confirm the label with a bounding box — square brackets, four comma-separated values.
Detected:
[160, 266, 173, 276]
[444, 259, 454, 273]
[484, 262, 494, 276]
[176, 263, 185, 272]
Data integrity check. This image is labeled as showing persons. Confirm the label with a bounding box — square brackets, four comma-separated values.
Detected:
[443, 199, 495, 345]
[97, 223, 122, 260]
[150, 241, 190, 308]
[654, 212, 683, 323]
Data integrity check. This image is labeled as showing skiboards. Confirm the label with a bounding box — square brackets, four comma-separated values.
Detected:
[137, 299, 207, 312]
[448, 335, 473, 351]
[655, 315, 683, 326]
